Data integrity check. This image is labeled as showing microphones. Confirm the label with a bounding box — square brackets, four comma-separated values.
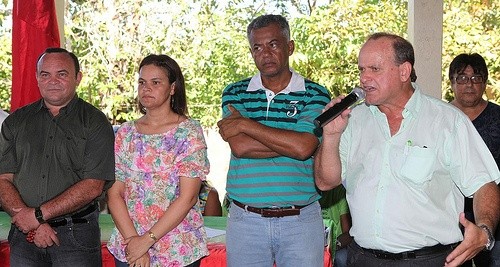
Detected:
[313, 86, 367, 128]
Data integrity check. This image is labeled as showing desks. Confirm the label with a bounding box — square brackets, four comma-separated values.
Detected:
[0, 210, 334, 266]
